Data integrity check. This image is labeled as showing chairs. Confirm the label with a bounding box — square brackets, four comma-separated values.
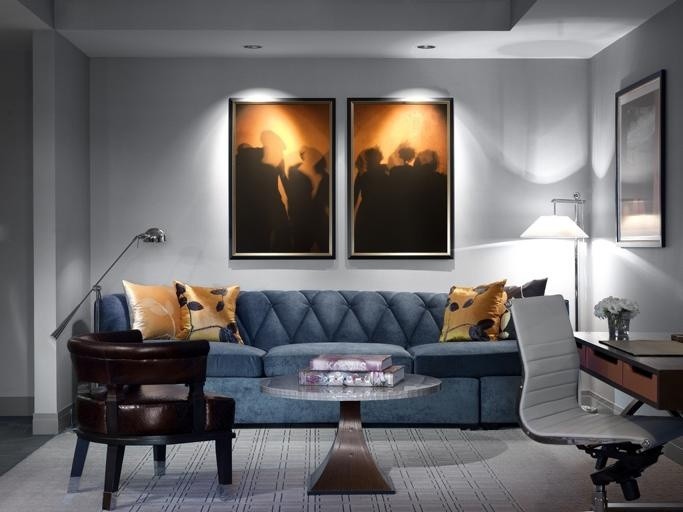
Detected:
[67, 329, 236, 511]
[504, 294, 682, 512]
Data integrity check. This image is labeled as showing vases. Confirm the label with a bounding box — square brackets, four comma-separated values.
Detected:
[608, 313, 632, 341]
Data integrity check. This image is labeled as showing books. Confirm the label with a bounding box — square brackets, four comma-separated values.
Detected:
[298, 352, 406, 388]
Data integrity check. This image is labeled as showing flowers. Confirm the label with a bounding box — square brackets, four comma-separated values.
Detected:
[592, 296, 644, 318]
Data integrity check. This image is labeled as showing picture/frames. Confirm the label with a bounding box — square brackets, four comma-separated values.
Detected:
[346, 96, 454, 261]
[610, 68, 669, 250]
[228, 97, 337, 260]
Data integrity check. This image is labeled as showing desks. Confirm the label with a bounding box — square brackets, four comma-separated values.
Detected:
[569, 331, 683, 471]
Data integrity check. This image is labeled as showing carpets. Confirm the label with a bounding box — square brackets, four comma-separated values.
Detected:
[0, 419, 682, 511]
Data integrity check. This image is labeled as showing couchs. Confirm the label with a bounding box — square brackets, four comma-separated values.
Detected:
[92, 289, 568, 430]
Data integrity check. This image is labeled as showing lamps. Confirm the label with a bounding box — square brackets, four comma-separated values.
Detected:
[49, 227, 168, 341]
[518, 191, 591, 333]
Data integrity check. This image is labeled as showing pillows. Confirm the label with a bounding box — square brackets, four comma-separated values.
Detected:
[497, 276, 549, 339]
[438, 278, 508, 344]
[120, 279, 182, 341]
[171, 279, 245, 346]
